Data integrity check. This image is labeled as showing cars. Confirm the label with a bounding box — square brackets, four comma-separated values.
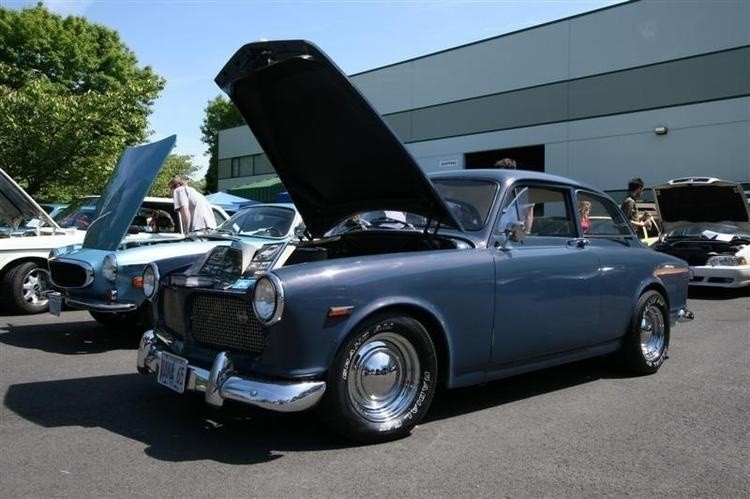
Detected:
[0, 133, 407, 340]
[649, 177, 750, 296]
[588, 203, 662, 247]
[136, 39, 693, 441]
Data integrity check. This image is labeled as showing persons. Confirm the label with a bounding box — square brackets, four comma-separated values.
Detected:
[494, 159, 535, 235]
[146, 208, 160, 226]
[168, 177, 217, 234]
[621, 177, 650, 238]
